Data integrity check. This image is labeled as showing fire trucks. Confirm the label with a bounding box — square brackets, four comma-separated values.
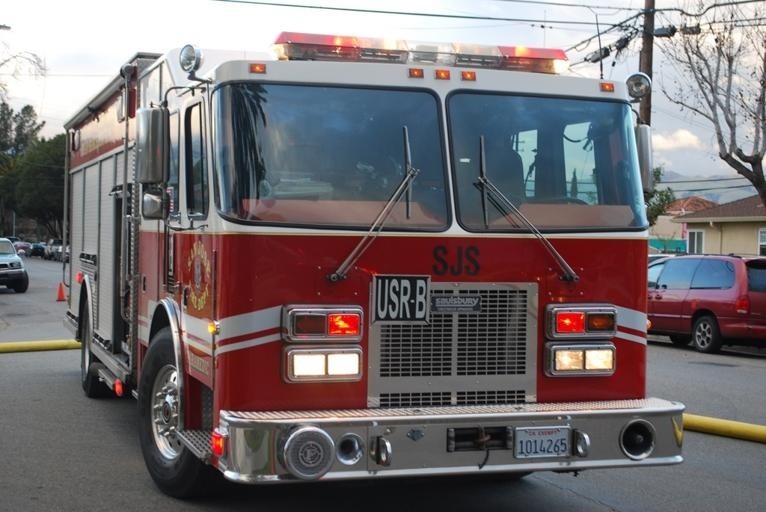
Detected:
[57, 28, 688, 499]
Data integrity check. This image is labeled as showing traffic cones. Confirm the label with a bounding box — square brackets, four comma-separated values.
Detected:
[57, 282, 67, 302]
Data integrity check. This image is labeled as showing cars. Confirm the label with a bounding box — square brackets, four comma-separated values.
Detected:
[7, 236, 70, 262]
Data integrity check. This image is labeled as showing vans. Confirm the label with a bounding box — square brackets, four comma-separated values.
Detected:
[646, 253, 765, 355]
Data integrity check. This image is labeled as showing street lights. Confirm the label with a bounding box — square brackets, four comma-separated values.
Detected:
[0, 24, 11, 30]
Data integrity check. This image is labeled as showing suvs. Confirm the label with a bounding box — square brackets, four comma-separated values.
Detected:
[0, 238, 28, 293]
